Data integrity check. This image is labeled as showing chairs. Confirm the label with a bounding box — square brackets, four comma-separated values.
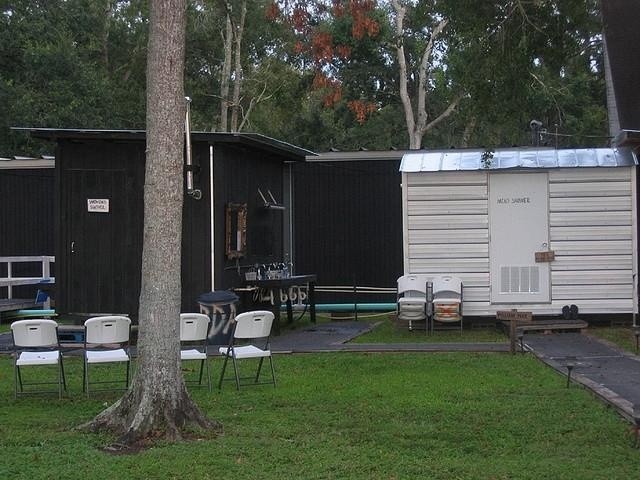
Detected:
[9, 318, 66, 402]
[82, 315, 132, 399]
[394, 273, 465, 337]
[180, 309, 276, 394]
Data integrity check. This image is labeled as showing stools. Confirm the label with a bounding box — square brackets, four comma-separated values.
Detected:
[197, 290, 240, 339]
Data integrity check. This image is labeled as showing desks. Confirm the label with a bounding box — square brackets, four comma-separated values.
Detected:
[244, 274, 318, 336]
